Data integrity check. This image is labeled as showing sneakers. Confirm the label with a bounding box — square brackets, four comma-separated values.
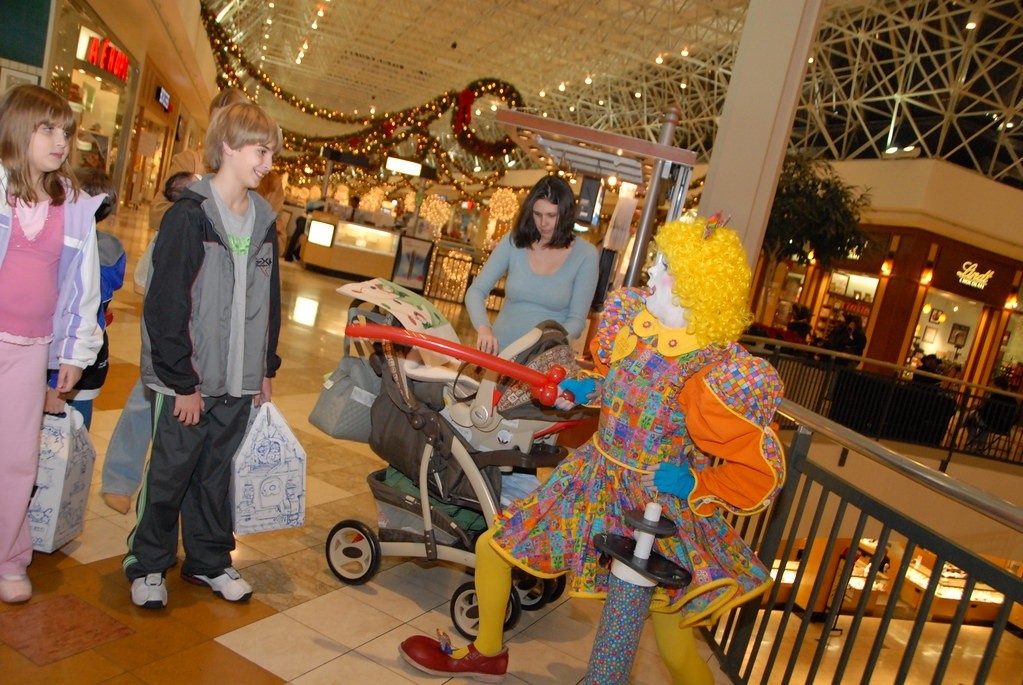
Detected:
[180, 567, 253, 602]
[131, 573, 168, 610]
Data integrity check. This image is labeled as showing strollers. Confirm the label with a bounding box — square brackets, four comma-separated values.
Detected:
[323, 297, 598, 644]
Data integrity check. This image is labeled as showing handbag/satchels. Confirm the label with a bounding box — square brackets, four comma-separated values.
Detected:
[310, 308, 391, 443]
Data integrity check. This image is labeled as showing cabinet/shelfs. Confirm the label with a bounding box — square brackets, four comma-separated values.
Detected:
[813, 291, 873, 339]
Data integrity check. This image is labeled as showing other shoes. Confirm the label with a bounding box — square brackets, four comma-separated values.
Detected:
[1, 575, 31, 603]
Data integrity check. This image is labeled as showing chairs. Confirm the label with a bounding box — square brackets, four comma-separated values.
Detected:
[965, 424, 1011, 459]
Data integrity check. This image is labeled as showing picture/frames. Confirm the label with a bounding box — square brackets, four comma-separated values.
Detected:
[828, 271, 850, 295]
[1002, 331, 1011, 346]
[921, 325, 939, 344]
[947, 323, 971, 347]
[928, 308, 944, 325]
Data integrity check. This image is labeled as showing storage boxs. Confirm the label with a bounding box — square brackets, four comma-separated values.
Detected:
[228, 392, 307, 535]
[26, 388, 96, 555]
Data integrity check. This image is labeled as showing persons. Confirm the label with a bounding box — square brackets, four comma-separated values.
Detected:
[165, 172, 199, 202]
[787, 304, 867, 368]
[913, 354, 942, 385]
[397, 214, 785, 685]
[124, 104, 282, 608]
[104, 89, 282, 514]
[0, 84, 104, 603]
[39, 168, 126, 429]
[964, 377, 1017, 449]
[466, 175, 600, 349]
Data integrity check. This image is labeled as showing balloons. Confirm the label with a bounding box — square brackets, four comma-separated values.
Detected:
[345, 323, 575, 406]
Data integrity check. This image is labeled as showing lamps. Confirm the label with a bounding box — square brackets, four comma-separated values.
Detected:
[882, 145, 921, 161]
